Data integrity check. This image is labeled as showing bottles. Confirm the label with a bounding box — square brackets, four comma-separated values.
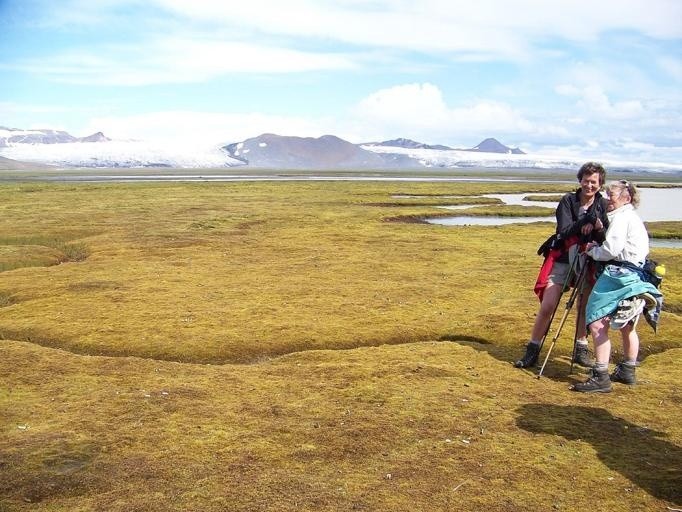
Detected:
[654, 264, 666, 278]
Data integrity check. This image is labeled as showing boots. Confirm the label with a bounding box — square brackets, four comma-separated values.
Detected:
[573, 342, 594, 367]
[515, 342, 541, 369]
[607, 361, 636, 385]
[574, 368, 613, 393]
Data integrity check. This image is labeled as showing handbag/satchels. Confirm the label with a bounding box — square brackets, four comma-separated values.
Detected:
[641, 260, 665, 292]
[537, 233, 558, 256]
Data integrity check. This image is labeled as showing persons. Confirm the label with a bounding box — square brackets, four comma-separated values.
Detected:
[568, 178, 649, 394]
[514, 162, 610, 369]
